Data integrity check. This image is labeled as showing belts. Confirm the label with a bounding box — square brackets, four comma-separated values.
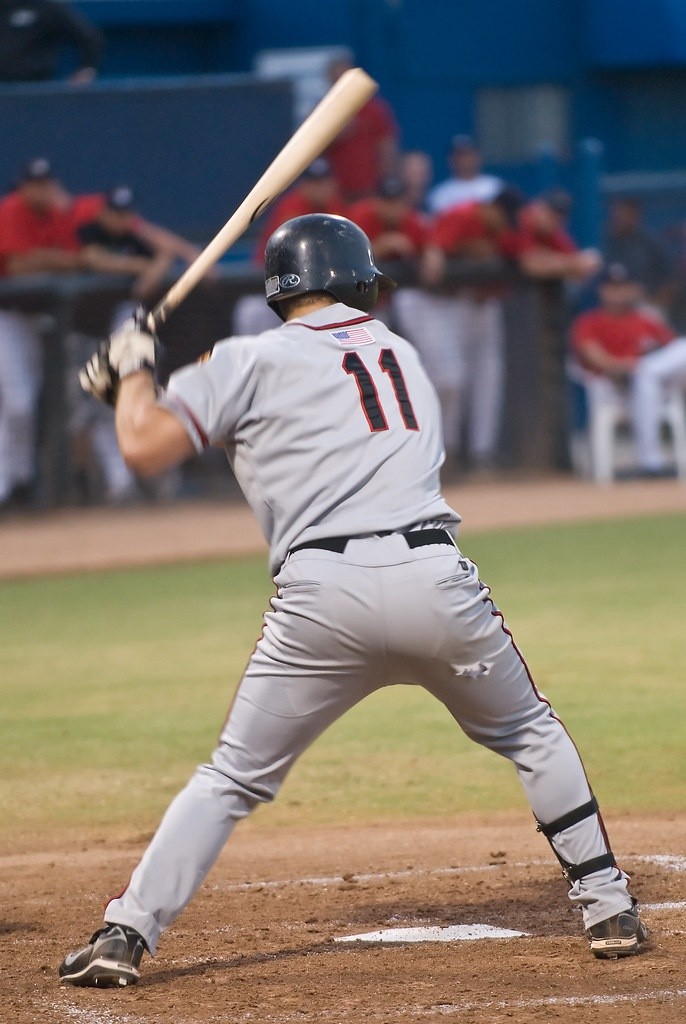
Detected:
[290, 530, 454, 555]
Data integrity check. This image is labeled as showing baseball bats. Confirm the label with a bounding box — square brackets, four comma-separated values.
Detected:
[94, 67, 379, 409]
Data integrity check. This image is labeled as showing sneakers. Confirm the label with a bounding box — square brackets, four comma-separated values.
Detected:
[588, 898, 647, 957]
[59, 921, 146, 989]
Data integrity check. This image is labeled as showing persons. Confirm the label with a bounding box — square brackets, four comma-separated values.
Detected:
[0, 59, 602, 281]
[571, 199, 686, 479]
[57, 213, 652, 989]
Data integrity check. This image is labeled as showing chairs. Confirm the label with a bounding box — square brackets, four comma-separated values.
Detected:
[561, 354, 686, 484]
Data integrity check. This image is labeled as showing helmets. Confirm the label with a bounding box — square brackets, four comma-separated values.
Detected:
[265, 213, 397, 311]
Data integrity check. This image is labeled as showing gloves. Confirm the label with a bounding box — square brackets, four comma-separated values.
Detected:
[77, 339, 116, 408]
[108, 305, 157, 378]
